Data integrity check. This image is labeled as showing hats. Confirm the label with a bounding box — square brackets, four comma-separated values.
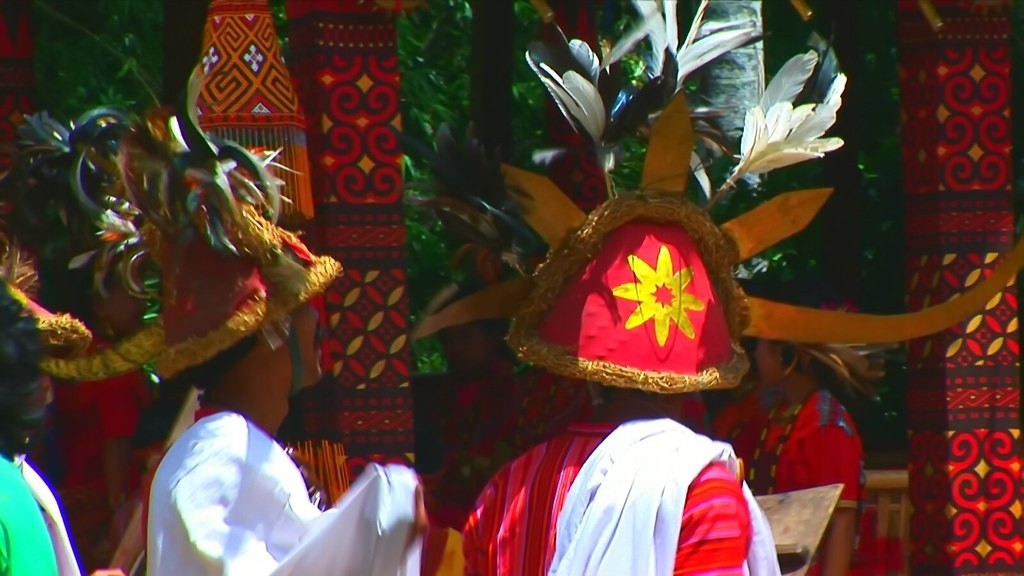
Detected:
[150, 186, 345, 381]
[31, 269, 165, 383]
[503, 188, 753, 393]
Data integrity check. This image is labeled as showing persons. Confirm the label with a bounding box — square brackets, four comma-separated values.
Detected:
[0, 289, 126, 576]
[725, 299, 869, 576]
[43, 250, 150, 576]
[145, 223, 428, 576]
[457, 189, 772, 576]
